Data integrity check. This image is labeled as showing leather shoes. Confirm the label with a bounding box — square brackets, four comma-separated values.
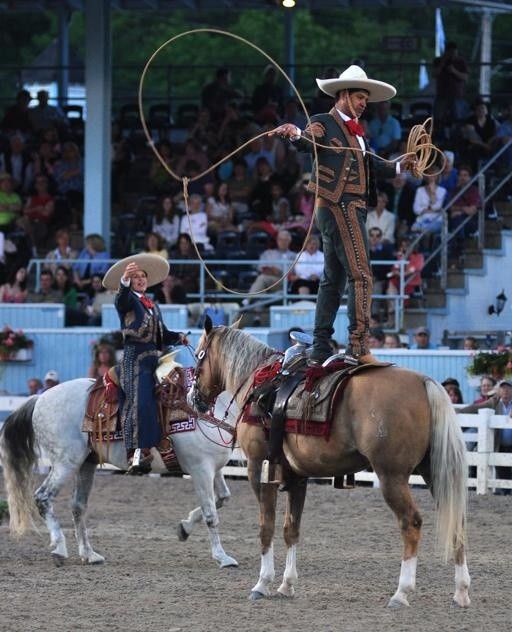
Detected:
[126, 448, 154, 466]
[307, 349, 334, 368]
[343, 347, 378, 367]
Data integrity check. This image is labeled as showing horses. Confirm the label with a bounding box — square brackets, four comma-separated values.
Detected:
[0, 376, 239, 570]
[186, 313, 471, 613]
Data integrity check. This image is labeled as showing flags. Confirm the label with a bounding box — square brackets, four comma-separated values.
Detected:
[417, 58, 429, 93]
[434, 8, 446, 58]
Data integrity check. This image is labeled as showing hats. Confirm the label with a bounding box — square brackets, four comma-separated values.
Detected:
[101, 252, 171, 292]
[44, 370, 59, 382]
[499, 380, 512, 388]
[314, 64, 397, 103]
[415, 326, 431, 338]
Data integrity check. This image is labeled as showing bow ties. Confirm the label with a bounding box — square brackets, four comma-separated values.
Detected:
[139, 295, 155, 310]
[343, 119, 366, 137]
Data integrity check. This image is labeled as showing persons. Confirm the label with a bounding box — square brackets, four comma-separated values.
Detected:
[428, 41, 470, 127]
[2, 86, 512, 348]
[198, 65, 241, 129]
[442, 348, 512, 496]
[350, 59, 376, 122]
[28, 343, 121, 392]
[261, 64, 418, 371]
[252, 64, 286, 118]
[314, 67, 339, 114]
[102, 253, 190, 475]
